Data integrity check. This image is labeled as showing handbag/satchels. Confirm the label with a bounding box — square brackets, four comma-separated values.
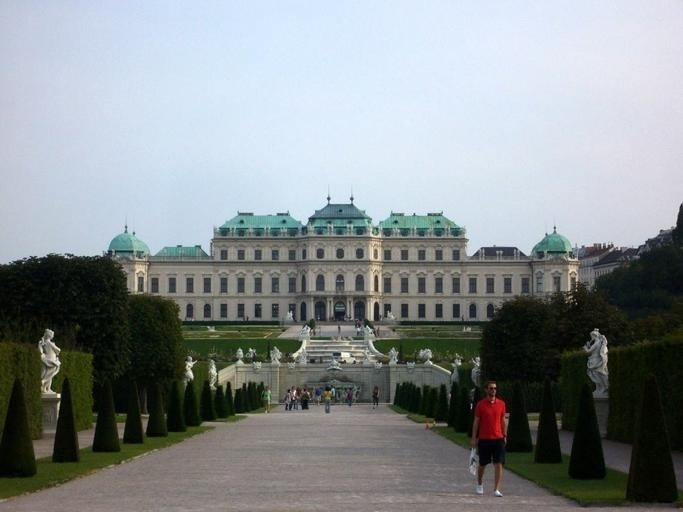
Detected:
[469, 453, 477, 475]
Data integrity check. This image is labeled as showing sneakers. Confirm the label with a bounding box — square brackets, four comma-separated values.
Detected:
[476, 481, 483, 494]
[494, 490, 502, 497]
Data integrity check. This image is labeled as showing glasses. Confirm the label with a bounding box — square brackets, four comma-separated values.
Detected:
[490, 387, 497, 389]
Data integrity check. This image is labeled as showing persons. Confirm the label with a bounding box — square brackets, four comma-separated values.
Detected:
[355, 319, 363, 336]
[184, 355, 198, 381]
[470, 380, 508, 498]
[38, 328, 62, 393]
[347, 388, 353, 406]
[372, 385, 379, 406]
[583, 327, 611, 395]
[261, 384, 273, 413]
[283, 383, 336, 414]
[472, 356, 480, 380]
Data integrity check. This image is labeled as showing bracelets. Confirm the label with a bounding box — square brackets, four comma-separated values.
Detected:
[504, 434, 508, 437]
